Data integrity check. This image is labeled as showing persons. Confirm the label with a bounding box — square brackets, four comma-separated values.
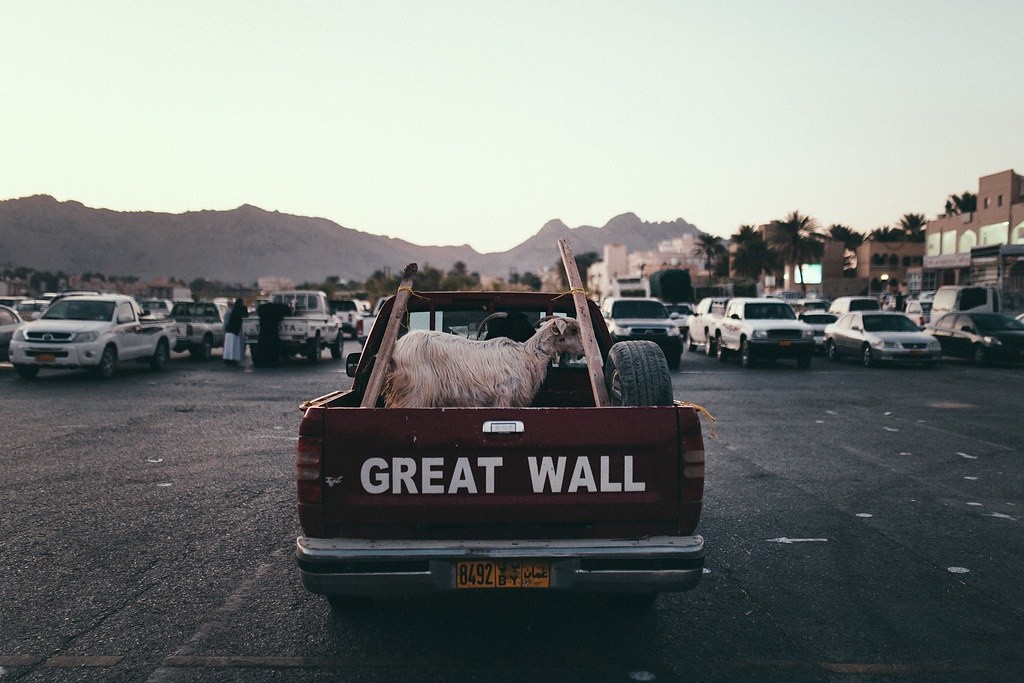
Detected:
[257, 302, 283, 368]
[895, 292, 907, 311]
[222, 298, 248, 365]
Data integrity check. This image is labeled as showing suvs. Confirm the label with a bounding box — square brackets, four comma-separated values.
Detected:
[599, 297, 684, 368]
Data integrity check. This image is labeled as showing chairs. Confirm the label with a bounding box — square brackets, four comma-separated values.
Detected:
[477, 313, 536, 346]
[180, 310, 192, 316]
[204, 310, 215, 317]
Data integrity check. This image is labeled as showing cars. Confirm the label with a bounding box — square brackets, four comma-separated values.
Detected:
[922, 311, 1024, 367]
[823, 311, 942, 369]
[664, 284, 1024, 357]
[0, 292, 176, 363]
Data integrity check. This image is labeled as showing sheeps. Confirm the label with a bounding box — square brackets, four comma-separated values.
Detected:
[381, 315, 586, 408]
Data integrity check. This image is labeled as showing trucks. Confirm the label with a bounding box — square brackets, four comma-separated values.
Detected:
[241, 289, 343, 367]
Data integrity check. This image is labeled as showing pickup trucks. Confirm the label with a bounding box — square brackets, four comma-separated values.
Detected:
[704, 297, 816, 369]
[8, 294, 179, 381]
[163, 297, 236, 362]
[296, 291, 705, 610]
[356, 296, 443, 352]
[327, 299, 365, 340]
